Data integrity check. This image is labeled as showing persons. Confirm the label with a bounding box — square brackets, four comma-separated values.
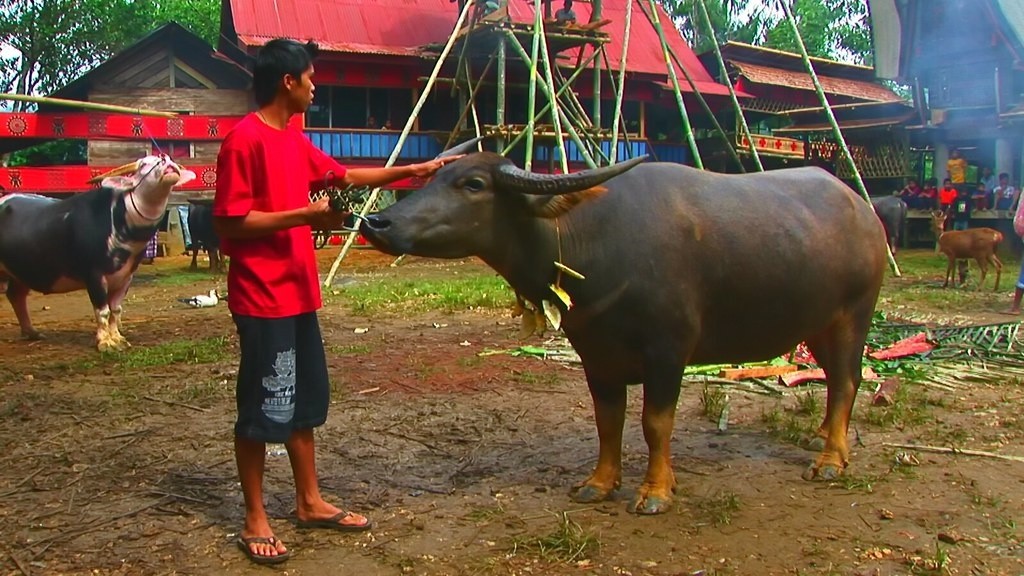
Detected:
[808, 149, 824, 169]
[946, 147, 972, 191]
[215, 36, 465, 564]
[365, 115, 379, 129]
[998, 190, 1024, 315]
[895, 164, 1019, 232]
[380, 119, 393, 131]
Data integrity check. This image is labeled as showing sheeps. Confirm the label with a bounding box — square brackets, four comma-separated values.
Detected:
[927, 209, 1005, 293]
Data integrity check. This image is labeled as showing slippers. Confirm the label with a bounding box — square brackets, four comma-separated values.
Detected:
[237, 536, 290, 565]
[294, 510, 373, 531]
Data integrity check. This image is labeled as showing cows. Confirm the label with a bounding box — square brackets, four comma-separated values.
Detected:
[869, 196, 908, 258]
[352, 134, 890, 518]
[0, 151, 197, 355]
